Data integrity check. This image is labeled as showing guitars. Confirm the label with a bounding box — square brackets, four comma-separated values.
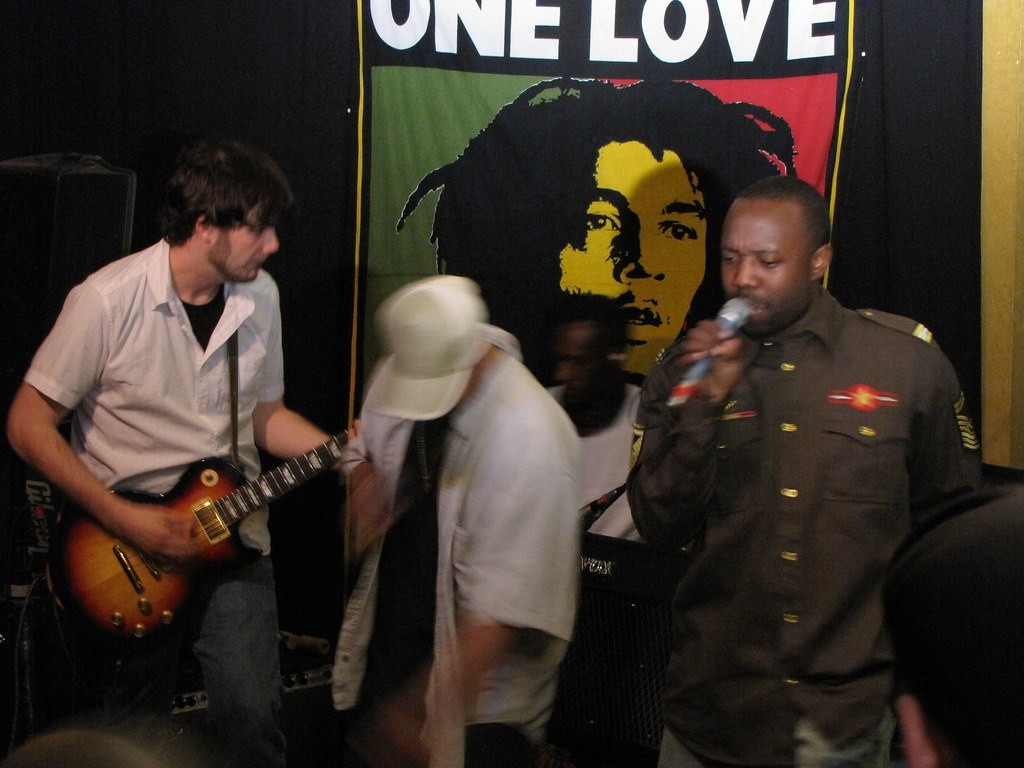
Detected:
[66, 417, 366, 644]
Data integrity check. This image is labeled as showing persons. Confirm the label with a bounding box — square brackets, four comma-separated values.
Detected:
[626, 176, 979, 768]
[6, 129, 645, 768]
[882, 480, 1024, 768]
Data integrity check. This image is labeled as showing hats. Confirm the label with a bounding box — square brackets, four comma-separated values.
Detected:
[366, 275, 490, 422]
[2, 730, 164, 768]
[883, 484, 1024, 768]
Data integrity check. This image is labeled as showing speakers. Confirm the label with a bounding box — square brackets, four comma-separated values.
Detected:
[546, 531, 686, 753]
[0, 152, 137, 375]
[163, 666, 359, 768]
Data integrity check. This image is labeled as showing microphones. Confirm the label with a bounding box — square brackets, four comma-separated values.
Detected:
[666, 298, 754, 406]
[577, 484, 626, 531]
